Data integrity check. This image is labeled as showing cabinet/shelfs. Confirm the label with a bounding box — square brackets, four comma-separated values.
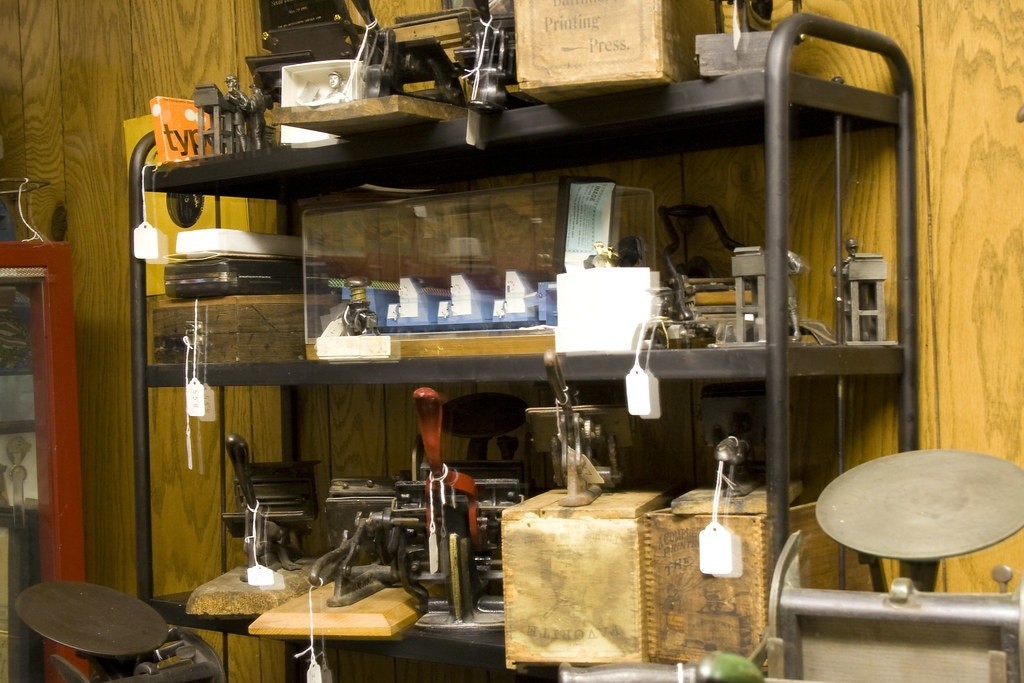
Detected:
[128, 13, 919, 683]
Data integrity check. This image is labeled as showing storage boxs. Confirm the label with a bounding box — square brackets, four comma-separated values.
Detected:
[154, 295, 341, 365]
[327, 477, 396, 549]
[282, 60, 360, 146]
[643, 478, 869, 672]
[502, 489, 666, 671]
[513, 0, 717, 105]
[302, 179, 656, 361]
